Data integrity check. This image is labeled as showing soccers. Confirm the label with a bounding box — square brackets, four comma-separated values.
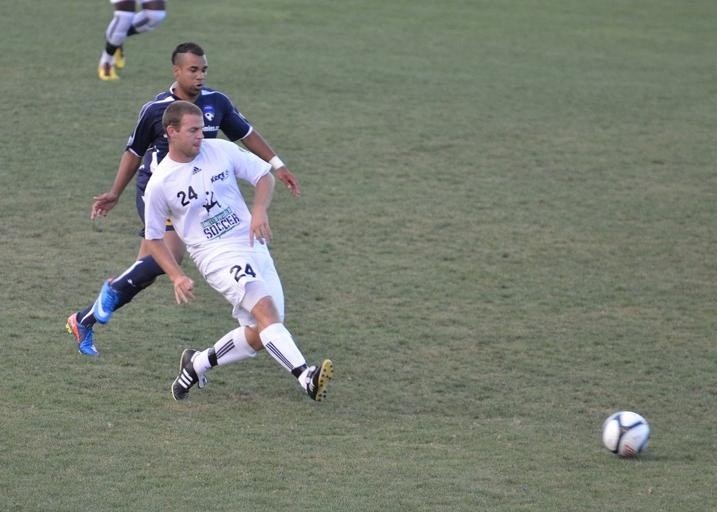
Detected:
[602, 411, 650, 457]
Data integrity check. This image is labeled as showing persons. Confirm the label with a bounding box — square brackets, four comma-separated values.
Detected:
[98, 0, 167, 82]
[142, 101, 334, 402]
[66, 42, 302, 357]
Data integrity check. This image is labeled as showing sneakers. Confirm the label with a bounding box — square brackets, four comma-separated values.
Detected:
[98, 62, 119, 84]
[305, 359, 334, 403]
[63, 312, 98, 359]
[171, 348, 208, 403]
[113, 47, 125, 71]
[93, 276, 119, 324]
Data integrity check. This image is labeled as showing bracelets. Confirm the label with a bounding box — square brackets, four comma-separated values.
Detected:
[268, 155, 284, 170]
